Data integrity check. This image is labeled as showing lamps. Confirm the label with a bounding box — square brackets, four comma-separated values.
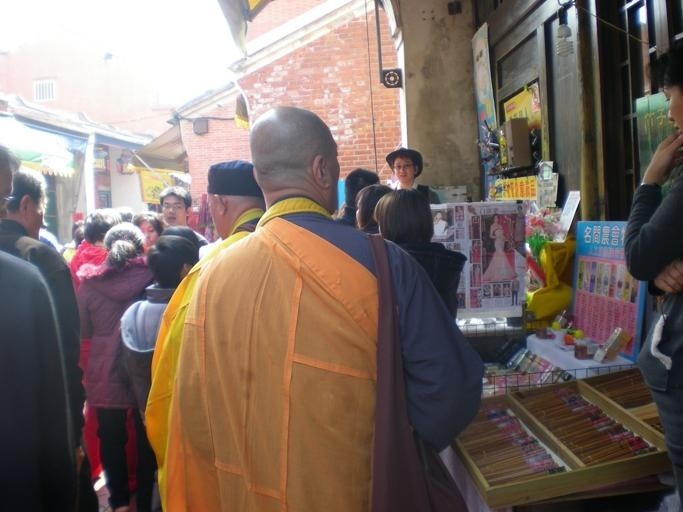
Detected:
[167, 112, 235, 136]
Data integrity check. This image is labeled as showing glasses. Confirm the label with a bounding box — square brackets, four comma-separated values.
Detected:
[395, 164, 414, 169]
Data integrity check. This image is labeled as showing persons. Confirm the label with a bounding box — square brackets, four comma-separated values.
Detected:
[622, 39, 683, 512]
[433, 206, 524, 308]
[0, 105, 487, 511]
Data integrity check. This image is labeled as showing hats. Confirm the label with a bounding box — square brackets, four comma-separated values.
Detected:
[386, 148, 422, 176]
[207, 160, 263, 196]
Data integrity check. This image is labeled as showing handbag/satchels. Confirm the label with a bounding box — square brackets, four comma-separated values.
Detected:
[372, 430, 468, 512]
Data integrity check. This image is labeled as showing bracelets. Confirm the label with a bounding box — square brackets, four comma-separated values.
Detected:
[638, 180, 661, 188]
